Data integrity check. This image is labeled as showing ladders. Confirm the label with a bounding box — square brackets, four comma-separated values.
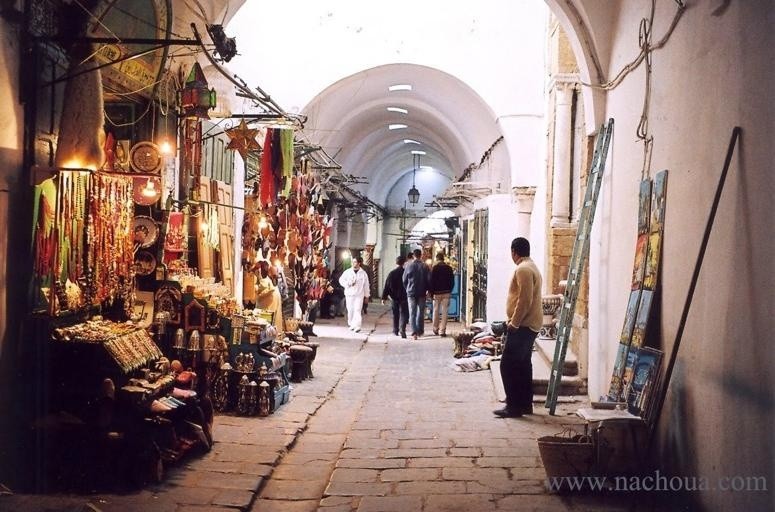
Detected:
[545, 117, 614, 415]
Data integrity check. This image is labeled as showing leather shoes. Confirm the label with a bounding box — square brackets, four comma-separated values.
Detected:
[494, 403, 532, 417]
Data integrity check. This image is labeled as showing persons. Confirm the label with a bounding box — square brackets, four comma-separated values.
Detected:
[381, 256, 409, 338]
[294, 257, 372, 337]
[253, 261, 283, 334]
[403, 252, 414, 269]
[431, 252, 454, 337]
[492, 238, 543, 418]
[401, 248, 431, 340]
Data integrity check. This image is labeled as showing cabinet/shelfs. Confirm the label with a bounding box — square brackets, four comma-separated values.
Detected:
[47, 318, 194, 489]
[430, 275, 461, 322]
[156, 261, 292, 414]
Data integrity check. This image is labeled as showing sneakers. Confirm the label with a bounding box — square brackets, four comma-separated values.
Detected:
[393, 327, 446, 339]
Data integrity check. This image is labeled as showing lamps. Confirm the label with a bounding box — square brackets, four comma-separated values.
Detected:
[408, 154, 420, 207]
